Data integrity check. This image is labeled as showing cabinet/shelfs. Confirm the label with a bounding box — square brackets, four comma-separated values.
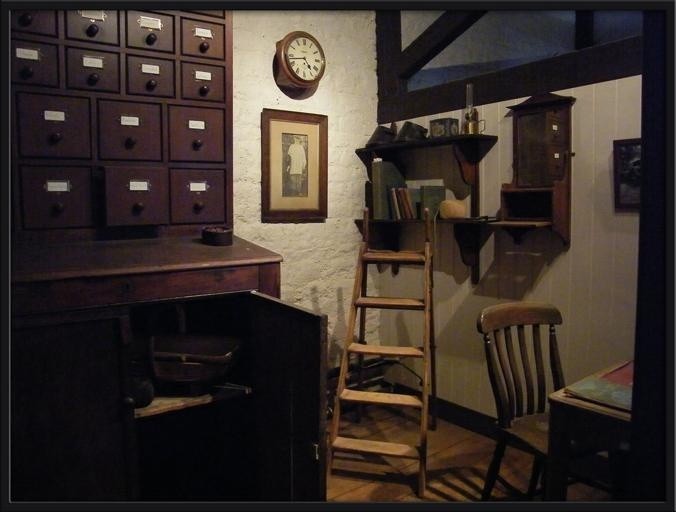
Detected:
[353, 135, 498, 283]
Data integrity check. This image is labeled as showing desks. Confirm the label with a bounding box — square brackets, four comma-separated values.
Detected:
[548, 359, 634, 502]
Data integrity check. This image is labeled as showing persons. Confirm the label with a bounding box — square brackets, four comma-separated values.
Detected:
[285, 135, 306, 196]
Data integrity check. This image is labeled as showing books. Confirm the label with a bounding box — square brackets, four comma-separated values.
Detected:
[390, 187, 444, 220]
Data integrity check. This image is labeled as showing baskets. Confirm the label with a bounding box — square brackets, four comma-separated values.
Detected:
[149, 304, 242, 397]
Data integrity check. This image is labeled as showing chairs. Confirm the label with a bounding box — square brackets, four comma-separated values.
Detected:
[476, 303, 565, 501]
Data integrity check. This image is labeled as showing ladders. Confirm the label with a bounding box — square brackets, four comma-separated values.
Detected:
[326, 207, 436, 497]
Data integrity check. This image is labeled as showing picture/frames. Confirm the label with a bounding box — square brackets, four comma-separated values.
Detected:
[613, 139, 642, 209]
